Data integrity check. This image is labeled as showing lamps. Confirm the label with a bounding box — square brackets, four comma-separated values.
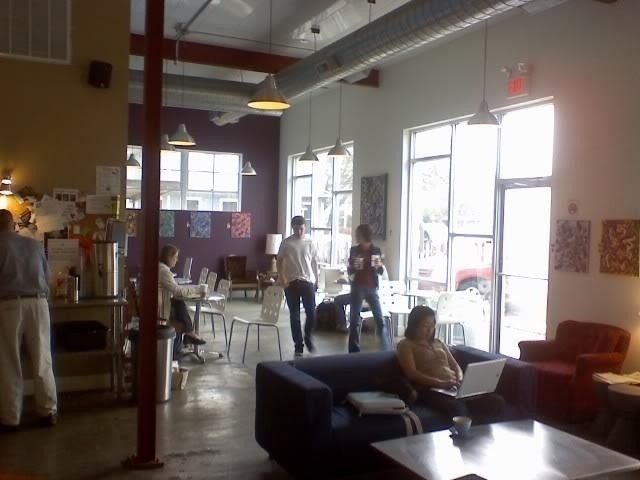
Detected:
[1, 183, 15, 199]
[465, 19, 503, 127]
[263, 233, 283, 273]
[244, 0, 293, 112]
[127, 25, 352, 176]
[1, 173, 13, 185]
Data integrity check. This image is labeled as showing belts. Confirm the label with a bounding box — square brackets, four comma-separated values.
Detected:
[5, 293, 46, 302]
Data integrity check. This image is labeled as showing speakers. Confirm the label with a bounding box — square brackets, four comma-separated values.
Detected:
[88, 60, 111, 90]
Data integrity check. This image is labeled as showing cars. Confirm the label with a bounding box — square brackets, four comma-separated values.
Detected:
[419, 259, 492, 312]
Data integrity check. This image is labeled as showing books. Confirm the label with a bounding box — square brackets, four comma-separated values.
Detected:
[592, 369, 640, 386]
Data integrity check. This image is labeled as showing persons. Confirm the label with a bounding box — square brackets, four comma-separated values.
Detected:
[397, 306, 488, 430]
[348, 223, 393, 353]
[0, 209, 60, 429]
[136, 245, 206, 361]
[275, 217, 320, 359]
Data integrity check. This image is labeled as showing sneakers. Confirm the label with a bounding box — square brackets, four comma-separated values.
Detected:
[183, 334, 206, 346]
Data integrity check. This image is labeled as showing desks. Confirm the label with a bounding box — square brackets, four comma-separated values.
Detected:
[592, 368, 639, 452]
[20, 296, 131, 402]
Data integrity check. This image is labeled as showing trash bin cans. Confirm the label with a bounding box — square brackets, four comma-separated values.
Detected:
[132, 325, 175, 403]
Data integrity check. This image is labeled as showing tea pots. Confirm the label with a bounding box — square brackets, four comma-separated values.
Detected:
[62, 272, 82, 304]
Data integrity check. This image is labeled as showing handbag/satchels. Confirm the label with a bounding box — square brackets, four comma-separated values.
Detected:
[348, 391, 409, 414]
[316, 299, 347, 332]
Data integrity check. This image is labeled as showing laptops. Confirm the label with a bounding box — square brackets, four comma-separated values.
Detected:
[429, 358, 507, 399]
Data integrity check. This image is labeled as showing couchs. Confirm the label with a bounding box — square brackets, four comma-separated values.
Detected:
[516, 311, 633, 425]
[251, 342, 539, 469]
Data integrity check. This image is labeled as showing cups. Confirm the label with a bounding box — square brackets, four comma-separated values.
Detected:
[355, 258, 364, 270]
[371, 255, 380, 267]
[200, 284, 208, 298]
[453, 414, 472, 435]
